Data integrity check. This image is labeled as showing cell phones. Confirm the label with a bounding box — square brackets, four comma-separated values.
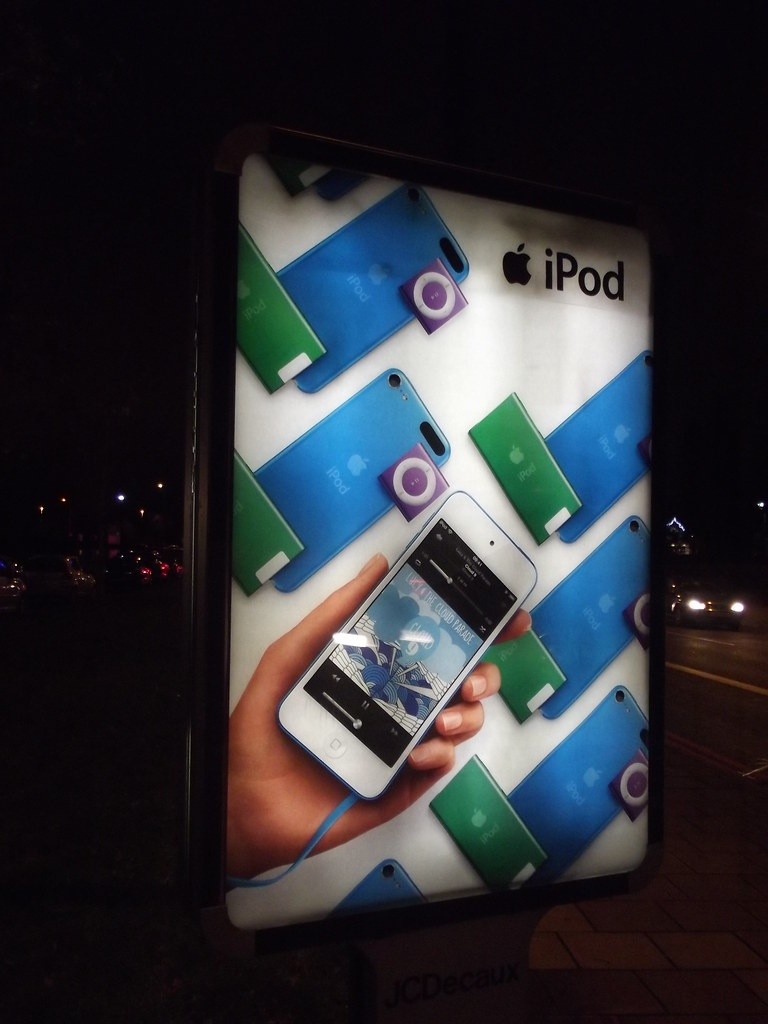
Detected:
[232, 179, 651, 919]
[277, 489, 537, 801]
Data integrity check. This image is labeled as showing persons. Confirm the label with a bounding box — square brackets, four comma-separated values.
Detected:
[229, 552, 532, 892]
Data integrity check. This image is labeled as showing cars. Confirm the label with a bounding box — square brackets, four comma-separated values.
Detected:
[22, 555, 95, 603]
[0, 557, 25, 613]
[666, 583, 745, 631]
[97, 545, 183, 591]
